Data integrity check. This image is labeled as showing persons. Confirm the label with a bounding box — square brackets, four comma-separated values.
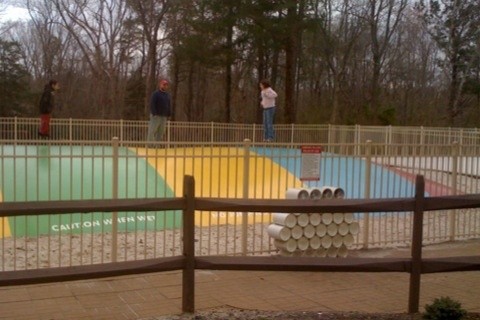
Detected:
[38, 79, 57, 137]
[145, 78, 172, 150]
[259, 81, 277, 142]
